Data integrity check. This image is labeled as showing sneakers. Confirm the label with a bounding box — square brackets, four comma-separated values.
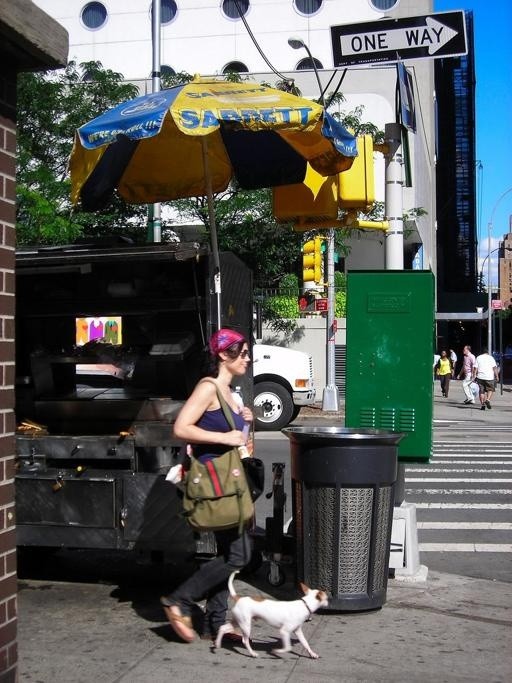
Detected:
[484, 399, 491, 409]
[442, 392, 445, 396]
[444, 396, 448, 398]
[479, 405, 485, 410]
[463, 399, 473, 404]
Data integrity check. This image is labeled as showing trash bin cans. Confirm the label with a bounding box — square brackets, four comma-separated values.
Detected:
[280, 426, 407, 610]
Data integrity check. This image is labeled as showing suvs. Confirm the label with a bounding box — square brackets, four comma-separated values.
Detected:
[503, 343, 512, 363]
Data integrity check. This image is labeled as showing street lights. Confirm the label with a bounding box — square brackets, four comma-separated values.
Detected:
[479, 246, 512, 293]
[286, 35, 341, 413]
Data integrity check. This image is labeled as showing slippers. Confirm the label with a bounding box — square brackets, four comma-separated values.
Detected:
[159, 594, 195, 643]
[200, 631, 254, 646]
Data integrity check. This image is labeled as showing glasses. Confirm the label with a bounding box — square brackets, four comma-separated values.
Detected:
[228, 348, 251, 358]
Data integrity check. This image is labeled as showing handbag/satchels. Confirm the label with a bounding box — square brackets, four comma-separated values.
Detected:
[180, 378, 256, 531]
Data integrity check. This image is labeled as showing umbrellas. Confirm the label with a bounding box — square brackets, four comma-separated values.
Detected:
[67, 75, 358, 331]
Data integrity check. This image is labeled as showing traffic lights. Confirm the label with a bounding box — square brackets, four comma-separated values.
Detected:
[300, 241, 323, 284]
[337, 132, 376, 211]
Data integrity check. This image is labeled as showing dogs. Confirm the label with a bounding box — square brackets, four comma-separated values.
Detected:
[212, 570, 330, 660]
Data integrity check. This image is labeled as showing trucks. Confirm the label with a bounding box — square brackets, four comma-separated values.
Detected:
[250, 335, 317, 431]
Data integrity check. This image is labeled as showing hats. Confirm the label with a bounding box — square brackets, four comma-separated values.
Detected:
[208, 328, 245, 355]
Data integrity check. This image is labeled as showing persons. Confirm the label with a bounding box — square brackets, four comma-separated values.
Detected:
[160, 329, 253, 642]
[437, 345, 500, 409]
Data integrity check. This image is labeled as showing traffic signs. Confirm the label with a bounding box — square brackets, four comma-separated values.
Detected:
[332, 10, 466, 70]
